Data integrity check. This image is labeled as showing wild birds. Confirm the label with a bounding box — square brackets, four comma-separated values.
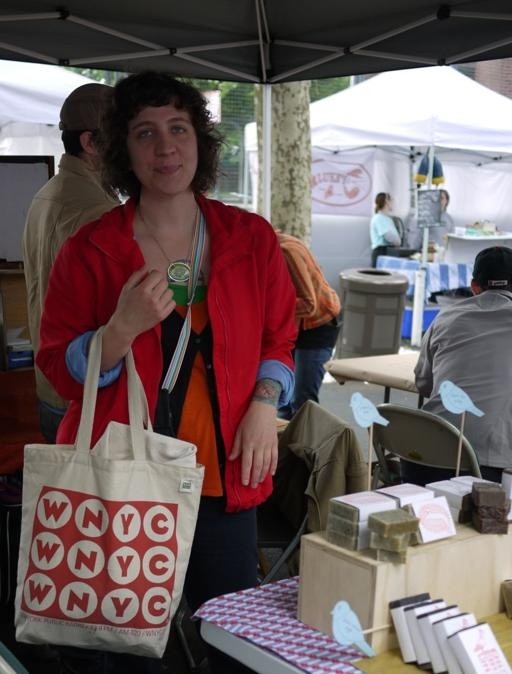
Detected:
[330, 601, 376, 657]
[437, 380, 485, 418]
[350, 392, 390, 429]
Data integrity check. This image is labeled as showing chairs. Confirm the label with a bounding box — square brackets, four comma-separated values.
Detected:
[367, 402, 487, 491]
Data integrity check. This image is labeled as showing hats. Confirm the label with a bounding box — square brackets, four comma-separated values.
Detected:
[59, 83, 115, 132]
[473, 246, 512, 287]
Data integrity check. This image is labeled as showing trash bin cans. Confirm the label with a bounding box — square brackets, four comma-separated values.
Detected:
[336, 267, 409, 358]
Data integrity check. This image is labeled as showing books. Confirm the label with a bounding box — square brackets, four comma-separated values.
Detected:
[6, 325, 35, 368]
[388, 592, 511, 674]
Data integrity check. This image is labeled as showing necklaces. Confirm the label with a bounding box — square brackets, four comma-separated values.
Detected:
[131, 202, 206, 284]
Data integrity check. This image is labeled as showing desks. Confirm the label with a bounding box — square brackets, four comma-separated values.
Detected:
[322, 351, 425, 409]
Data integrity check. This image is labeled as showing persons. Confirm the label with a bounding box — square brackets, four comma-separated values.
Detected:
[22, 80, 125, 446]
[408, 188, 455, 254]
[394, 245, 511, 486]
[273, 225, 345, 425]
[36, 66, 305, 674]
[368, 190, 402, 269]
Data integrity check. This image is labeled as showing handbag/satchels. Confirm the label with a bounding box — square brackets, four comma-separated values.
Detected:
[14, 325, 207, 660]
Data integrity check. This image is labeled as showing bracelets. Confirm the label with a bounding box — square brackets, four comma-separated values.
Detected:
[251, 394, 279, 407]
[257, 378, 282, 394]
